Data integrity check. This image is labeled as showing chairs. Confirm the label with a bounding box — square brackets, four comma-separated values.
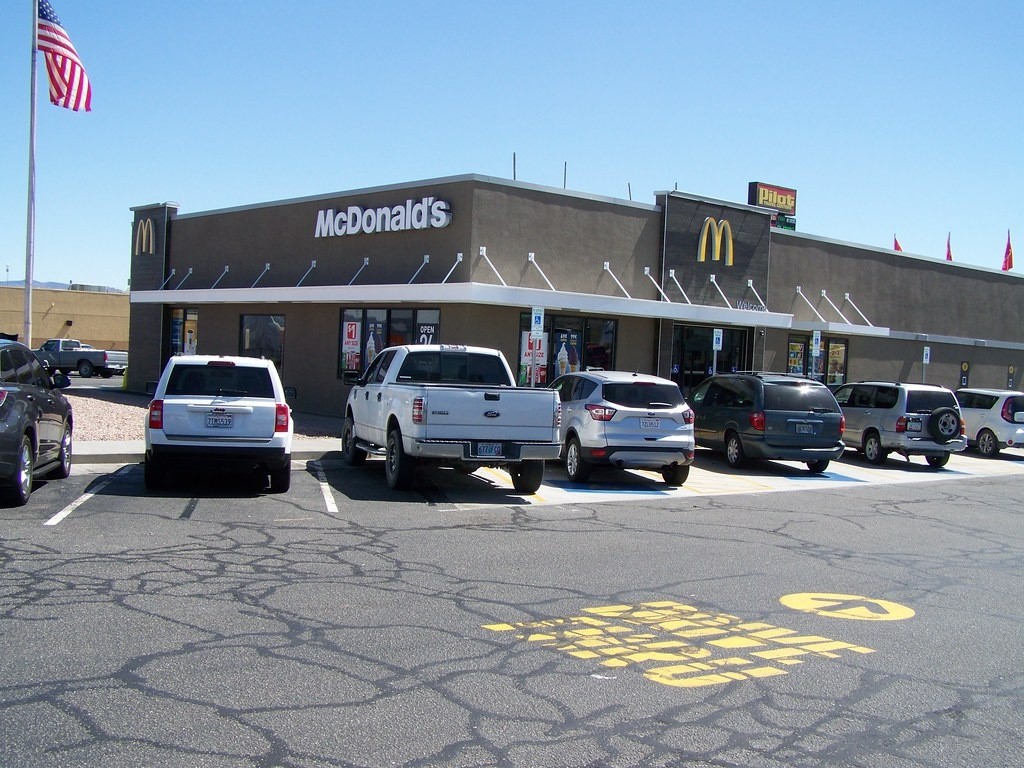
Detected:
[239, 375, 262, 396]
[181, 371, 207, 393]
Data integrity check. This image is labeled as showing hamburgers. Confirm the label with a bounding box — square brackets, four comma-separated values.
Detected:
[828, 359, 843, 383]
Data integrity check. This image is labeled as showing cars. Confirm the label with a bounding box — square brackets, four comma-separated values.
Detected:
[0, 337, 74, 506]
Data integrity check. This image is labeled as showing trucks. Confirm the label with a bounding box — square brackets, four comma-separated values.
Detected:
[29, 338, 130, 379]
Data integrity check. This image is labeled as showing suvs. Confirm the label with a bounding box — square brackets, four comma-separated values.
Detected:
[831, 380, 968, 469]
[545, 368, 696, 486]
[953, 386, 1024, 458]
[145, 352, 298, 493]
[686, 369, 846, 473]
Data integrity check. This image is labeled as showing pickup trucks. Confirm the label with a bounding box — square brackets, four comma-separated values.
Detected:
[342, 343, 563, 495]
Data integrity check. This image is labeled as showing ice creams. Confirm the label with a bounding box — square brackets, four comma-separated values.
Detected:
[557, 343, 579, 373]
[367, 331, 375, 361]
